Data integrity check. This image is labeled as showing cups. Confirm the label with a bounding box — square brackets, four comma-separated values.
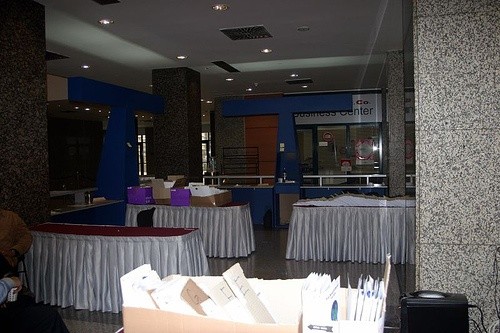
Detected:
[6, 287, 17, 302]
[281, 168, 287, 182]
[278, 178, 283, 183]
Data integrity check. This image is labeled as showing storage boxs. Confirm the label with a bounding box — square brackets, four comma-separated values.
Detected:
[121, 274, 308, 332]
[127, 186, 156, 205]
[301, 285, 387, 333]
[191, 189, 232, 207]
[170, 187, 192, 206]
[151, 178, 187, 205]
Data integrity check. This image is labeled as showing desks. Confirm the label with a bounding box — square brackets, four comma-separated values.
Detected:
[123, 202, 256, 258]
[17, 221, 211, 314]
[286, 197, 415, 265]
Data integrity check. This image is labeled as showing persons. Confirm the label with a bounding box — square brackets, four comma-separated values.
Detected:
[0, 276, 71, 333]
[0, 209, 34, 305]
